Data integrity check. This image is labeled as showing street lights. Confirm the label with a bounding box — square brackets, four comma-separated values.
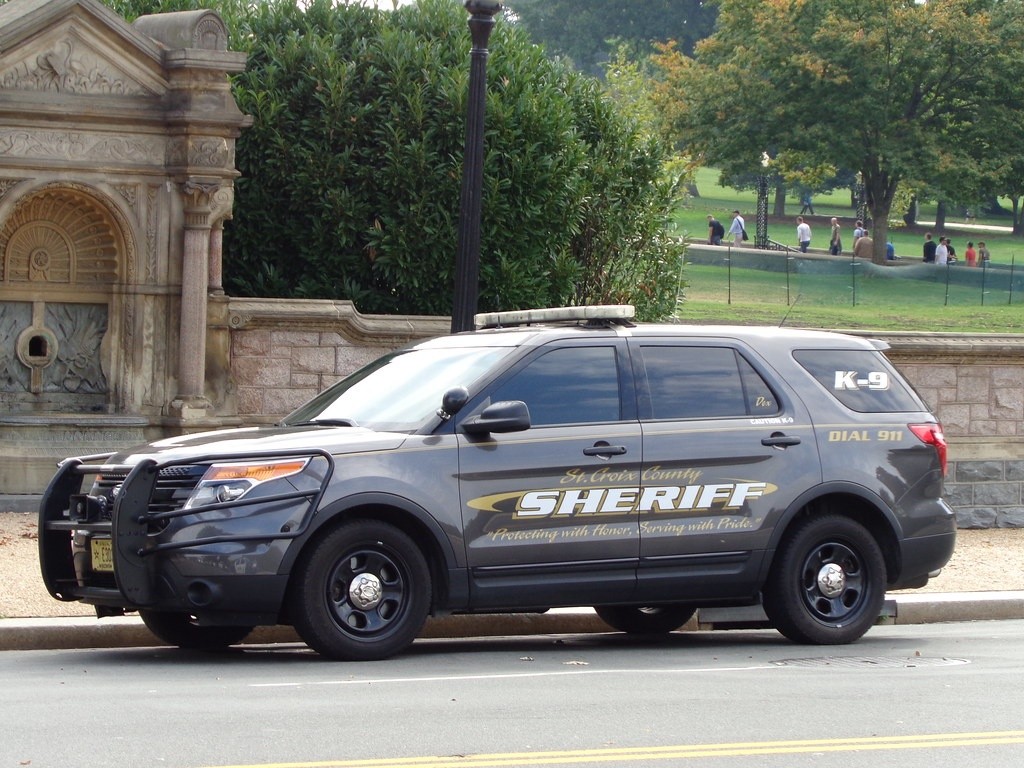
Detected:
[753, 151, 769, 249]
[852, 170, 868, 229]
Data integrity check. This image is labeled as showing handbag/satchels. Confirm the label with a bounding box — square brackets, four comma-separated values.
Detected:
[742, 229, 748, 240]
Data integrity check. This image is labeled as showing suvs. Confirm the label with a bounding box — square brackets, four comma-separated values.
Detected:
[37, 303, 955, 662]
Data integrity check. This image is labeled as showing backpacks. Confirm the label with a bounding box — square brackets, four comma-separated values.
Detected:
[719, 223, 725, 239]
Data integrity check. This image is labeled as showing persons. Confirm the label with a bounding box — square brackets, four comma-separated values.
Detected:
[829, 218, 842, 255]
[797, 217, 812, 253]
[965, 241, 976, 267]
[978, 242, 991, 267]
[934, 236, 947, 264]
[707, 214, 721, 245]
[946, 237, 956, 262]
[853, 230, 872, 258]
[886, 241, 894, 260]
[853, 220, 865, 250]
[923, 233, 937, 263]
[727, 209, 744, 246]
[800, 195, 814, 214]
